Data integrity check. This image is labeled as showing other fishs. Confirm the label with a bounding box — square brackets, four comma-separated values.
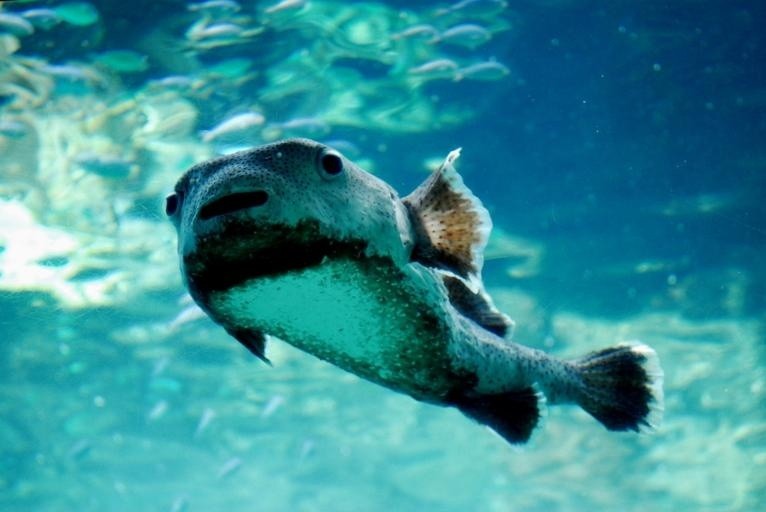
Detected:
[0, 0, 511, 328]
